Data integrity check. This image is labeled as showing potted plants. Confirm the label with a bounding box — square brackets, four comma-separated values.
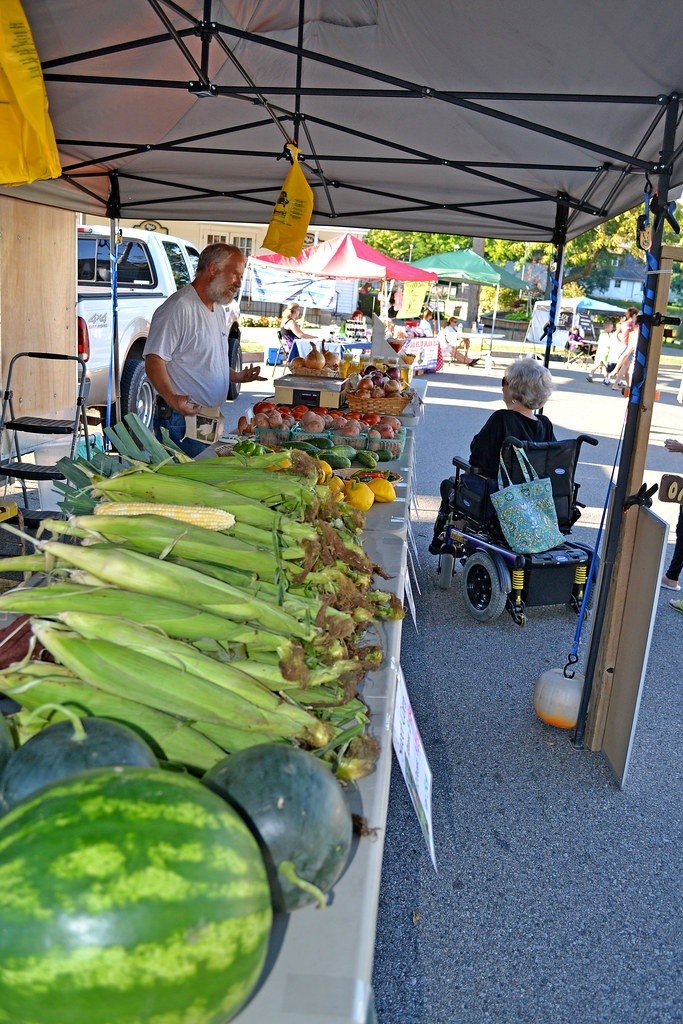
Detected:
[240, 340, 265, 363]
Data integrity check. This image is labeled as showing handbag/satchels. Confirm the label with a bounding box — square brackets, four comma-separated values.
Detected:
[490, 444, 566, 555]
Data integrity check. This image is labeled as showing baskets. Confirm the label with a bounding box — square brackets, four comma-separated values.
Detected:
[345, 388, 413, 415]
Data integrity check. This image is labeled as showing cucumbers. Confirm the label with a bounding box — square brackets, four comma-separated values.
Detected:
[274, 437, 394, 468]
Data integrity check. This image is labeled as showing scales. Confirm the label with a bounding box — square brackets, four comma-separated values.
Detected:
[274, 374, 350, 409]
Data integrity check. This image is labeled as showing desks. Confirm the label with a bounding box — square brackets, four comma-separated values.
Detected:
[584, 341, 599, 345]
[220, 529, 408, 1024]
[403, 337, 439, 376]
[194, 427, 414, 539]
[449, 332, 506, 370]
[288, 339, 372, 363]
[343, 377, 428, 427]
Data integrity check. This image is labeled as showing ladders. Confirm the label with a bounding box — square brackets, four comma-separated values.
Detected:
[0, 351, 91, 558]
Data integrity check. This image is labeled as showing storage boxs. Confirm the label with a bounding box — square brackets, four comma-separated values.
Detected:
[253, 425, 294, 445]
[291, 426, 330, 440]
[367, 435, 406, 460]
[524, 542, 588, 607]
[397, 425, 408, 437]
[328, 430, 368, 450]
[264, 346, 284, 366]
[295, 421, 300, 427]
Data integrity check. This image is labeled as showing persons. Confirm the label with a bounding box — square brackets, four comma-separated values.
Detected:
[419, 309, 433, 338]
[280, 303, 319, 357]
[586, 306, 643, 390]
[568, 326, 597, 360]
[338, 310, 364, 338]
[437, 316, 481, 367]
[468, 358, 558, 476]
[660, 438, 683, 613]
[142, 243, 269, 464]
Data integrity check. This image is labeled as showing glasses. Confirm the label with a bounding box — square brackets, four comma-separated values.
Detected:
[451, 320, 457, 324]
[502, 376, 509, 388]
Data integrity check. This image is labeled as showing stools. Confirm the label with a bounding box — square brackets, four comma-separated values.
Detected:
[0, 499, 28, 556]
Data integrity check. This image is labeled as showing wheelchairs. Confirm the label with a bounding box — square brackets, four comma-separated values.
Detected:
[436, 435, 603, 622]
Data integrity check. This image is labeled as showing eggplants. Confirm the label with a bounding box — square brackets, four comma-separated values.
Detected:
[360, 363, 401, 386]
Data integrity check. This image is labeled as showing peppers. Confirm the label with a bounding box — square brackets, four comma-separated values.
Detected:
[233, 437, 265, 456]
[329, 468, 397, 482]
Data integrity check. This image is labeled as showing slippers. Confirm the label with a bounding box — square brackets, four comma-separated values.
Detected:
[661, 577, 681, 591]
[669, 598, 683, 614]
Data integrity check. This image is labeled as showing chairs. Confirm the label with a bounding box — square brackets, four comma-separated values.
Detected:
[272, 330, 288, 377]
[564, 341, 591, 370]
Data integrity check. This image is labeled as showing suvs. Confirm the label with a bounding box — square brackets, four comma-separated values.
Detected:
[77, 228, 243, 448]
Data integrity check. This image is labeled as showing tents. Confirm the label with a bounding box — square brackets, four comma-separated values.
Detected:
[522, 295, 627, 369]
[237, 232, 440, 336]
[386, 249, 538, 366]
[0, 0, 683, 750]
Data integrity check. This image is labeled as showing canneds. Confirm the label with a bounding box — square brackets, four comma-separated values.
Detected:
[345, 320, 366, 339]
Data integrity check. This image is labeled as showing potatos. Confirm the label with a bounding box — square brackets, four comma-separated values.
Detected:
[238, 409, 403, 458]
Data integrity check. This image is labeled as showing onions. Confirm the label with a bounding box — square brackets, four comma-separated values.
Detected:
[291, 339, 339, 369]
[350, 372, 404, 398]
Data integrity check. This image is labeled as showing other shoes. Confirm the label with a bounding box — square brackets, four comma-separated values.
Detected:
[602, 380, 612, 387]
[611, 386, 622, 391]
[469, 358, 480, 366]
[586, 375, 593, 383]
[618, 381, 627, 387]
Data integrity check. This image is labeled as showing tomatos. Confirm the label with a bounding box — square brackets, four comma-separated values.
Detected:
[253, 402, 382, 424]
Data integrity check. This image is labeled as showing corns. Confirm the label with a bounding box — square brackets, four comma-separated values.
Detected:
[0, 451, 392, 780]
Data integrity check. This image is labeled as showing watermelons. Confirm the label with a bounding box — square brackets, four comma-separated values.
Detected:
[0, 716, 352, 1024]
